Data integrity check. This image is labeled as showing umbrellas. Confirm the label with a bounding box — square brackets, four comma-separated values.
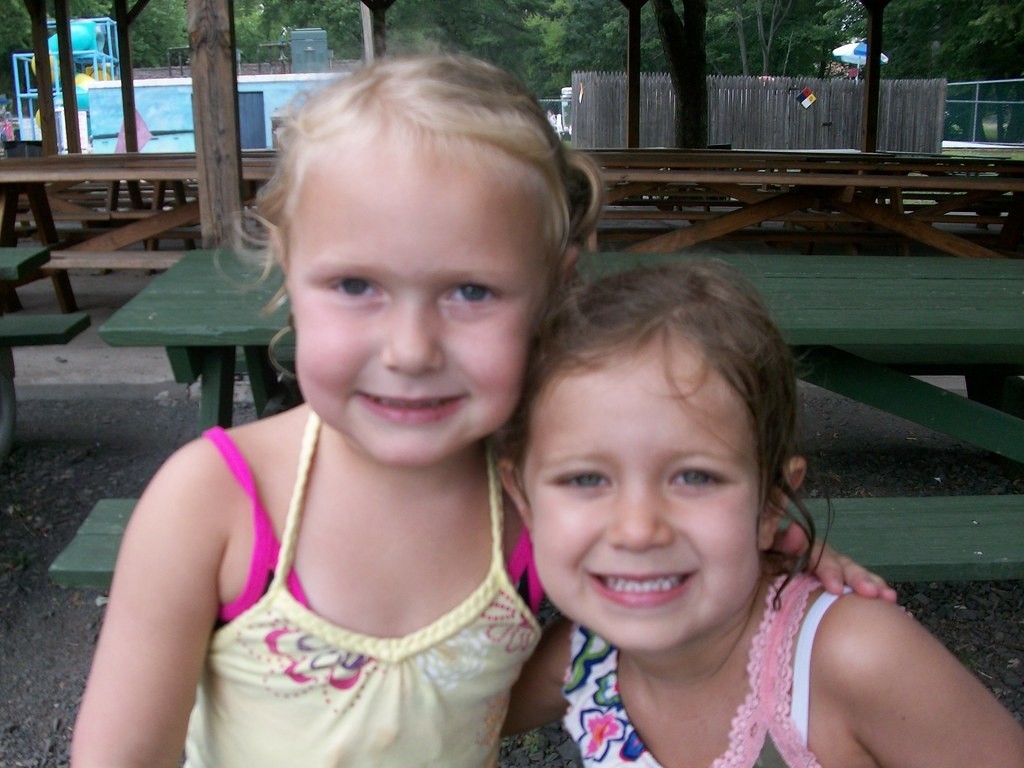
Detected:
[832, 43, 889, 79]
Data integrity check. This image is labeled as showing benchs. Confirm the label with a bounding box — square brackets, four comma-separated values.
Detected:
[67, 183, 155, 190]
[592, 229, 904, 246]
[49, 493, 1024, 592]
[41, 249, 190, 270]
[901, 194, 970, 199]
[0, 311, 92, 346]
[59, 190, 176, 197]
[904, 203, 1012, 211]
[70, 198, 196, 206]
[911, 214, 1008, 223]
[16, 209, 164, 221]
[598, 209, 863, 221]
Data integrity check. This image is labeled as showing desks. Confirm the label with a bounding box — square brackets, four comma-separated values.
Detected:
[98, 248, 1024, 465]
[0, 246, 57, 460]
[0, 155, 1024, 258]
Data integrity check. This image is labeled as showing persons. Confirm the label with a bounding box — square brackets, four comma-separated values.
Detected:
[497, 261, 1024, 768]
[63, 53, 900, 768]
[547, 110, 557, 124]
